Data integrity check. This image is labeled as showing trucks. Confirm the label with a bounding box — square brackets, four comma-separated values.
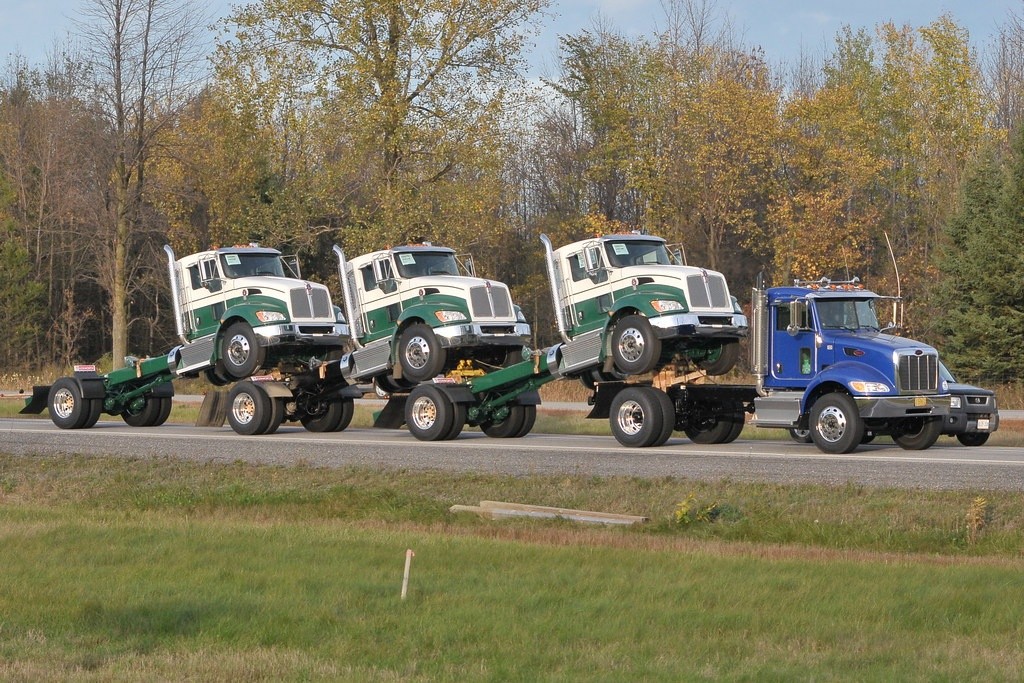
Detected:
[584, 274, 951, 456]
[372, 232, 747, 442]
[16, 243, 347, 430]
[192, 247, 534, 435]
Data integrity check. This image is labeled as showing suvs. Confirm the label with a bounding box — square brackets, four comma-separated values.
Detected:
[937, 357, 1001, 447]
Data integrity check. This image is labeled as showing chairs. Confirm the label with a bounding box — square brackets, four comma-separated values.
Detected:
[822, 302, 838, 327]
[777, 306, 790, 331]
